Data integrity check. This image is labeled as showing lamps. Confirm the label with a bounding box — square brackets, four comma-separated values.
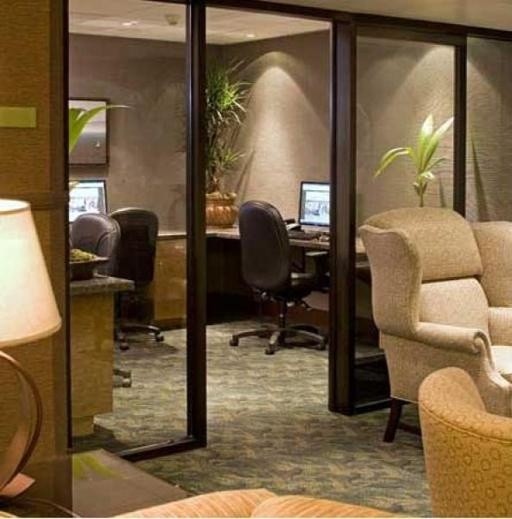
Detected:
[1, 195, 64, 508]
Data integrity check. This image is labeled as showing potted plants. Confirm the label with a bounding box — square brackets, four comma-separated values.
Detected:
[203, 53, 257, 230]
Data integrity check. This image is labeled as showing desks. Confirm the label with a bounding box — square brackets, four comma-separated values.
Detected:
[150, 227, 332, 334]
[0, 443, 198, 519]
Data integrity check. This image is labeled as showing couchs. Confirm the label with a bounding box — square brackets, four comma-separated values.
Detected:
[413, 364, 512, 518]
[101, 486, 412, 519]
[356, 206, 511, 448]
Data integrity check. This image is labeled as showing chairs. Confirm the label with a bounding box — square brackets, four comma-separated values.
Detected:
[227, 199, 328, 355]
[72, 212, 133, 388]
[109, 206, 165, 352]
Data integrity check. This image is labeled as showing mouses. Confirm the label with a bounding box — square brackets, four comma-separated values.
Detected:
[320, 235, 331, 242]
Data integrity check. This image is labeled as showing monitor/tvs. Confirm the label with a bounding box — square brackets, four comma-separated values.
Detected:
[298, 180, 330, 226]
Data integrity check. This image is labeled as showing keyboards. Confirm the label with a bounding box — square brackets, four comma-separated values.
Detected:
[287, 228, 318, 239]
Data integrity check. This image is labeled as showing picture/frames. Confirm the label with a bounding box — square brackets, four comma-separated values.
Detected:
[69, 97, 110, 166]
